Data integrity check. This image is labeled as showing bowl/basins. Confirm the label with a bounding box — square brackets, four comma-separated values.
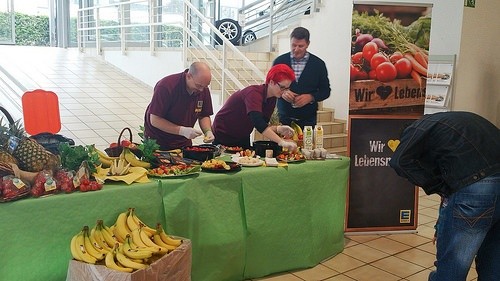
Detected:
[252, 140, 282, 158]
[181, 143, 219, 160]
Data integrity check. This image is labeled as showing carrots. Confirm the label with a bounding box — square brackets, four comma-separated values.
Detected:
[403, 43, 429, 88]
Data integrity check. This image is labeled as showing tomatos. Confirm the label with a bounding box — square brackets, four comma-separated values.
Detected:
[350, 42, 413, 83]
[0, 169, 102, 201]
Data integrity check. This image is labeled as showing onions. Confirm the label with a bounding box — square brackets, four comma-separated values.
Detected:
[354, 28, 388, 49]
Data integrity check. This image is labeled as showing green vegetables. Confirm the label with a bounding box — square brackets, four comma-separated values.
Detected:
[57, 142, 100, 174]
[173, 165, 201, 175]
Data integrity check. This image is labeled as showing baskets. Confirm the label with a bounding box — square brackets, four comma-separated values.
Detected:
[105, 128, 141, 158]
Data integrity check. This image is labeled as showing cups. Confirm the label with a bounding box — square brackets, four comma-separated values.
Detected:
[302, 147, 327, 160]
[291, 95, 301, 108]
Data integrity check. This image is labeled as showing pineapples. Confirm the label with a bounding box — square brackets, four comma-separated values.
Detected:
[0, 116, 51, 172]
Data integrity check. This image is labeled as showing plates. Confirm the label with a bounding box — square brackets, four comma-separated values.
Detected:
[148, 169, 203, 177]
[232, 157, 263, 166]
[276, 156, 306, 163]
[224, 145, 247, 152]
[231, 154, 260, 159]
[201, 161, 242, 172]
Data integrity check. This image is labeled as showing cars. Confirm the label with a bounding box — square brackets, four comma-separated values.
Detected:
[238, 0, 312, 46]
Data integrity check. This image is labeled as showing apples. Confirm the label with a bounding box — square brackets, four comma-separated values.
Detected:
[110, 143, 118, 147]
[122, 140, 137, 149]
[298, 135, 303, 140]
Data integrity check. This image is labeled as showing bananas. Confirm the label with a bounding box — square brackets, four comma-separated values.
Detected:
[70, 207, 182, 273]
[95, 149, 120, 168]
[120, 147, 150, 168]
[291, 121, 302, 141]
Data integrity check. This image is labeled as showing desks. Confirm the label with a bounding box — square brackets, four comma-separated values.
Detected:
[0, 151, 350, 281]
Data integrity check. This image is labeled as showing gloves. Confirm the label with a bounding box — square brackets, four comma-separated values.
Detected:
[203, 131, 216, 143]
[178, 126, 202, 140]
[277, 126, 294, 137]
[278, 139, 297, 151]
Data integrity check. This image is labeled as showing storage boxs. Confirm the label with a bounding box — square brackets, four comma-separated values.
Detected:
[65, 234, 192, 281]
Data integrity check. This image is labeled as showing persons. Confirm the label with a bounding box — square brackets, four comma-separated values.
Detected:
[389, 111, 500, 281]
[145, 62, 215, 150]
[272, 27, 331, 132]
[211, 64, 297, 151]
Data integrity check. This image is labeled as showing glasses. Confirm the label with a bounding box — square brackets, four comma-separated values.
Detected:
[191, 78, 208, 90]
[277, 82, 290, 91]
